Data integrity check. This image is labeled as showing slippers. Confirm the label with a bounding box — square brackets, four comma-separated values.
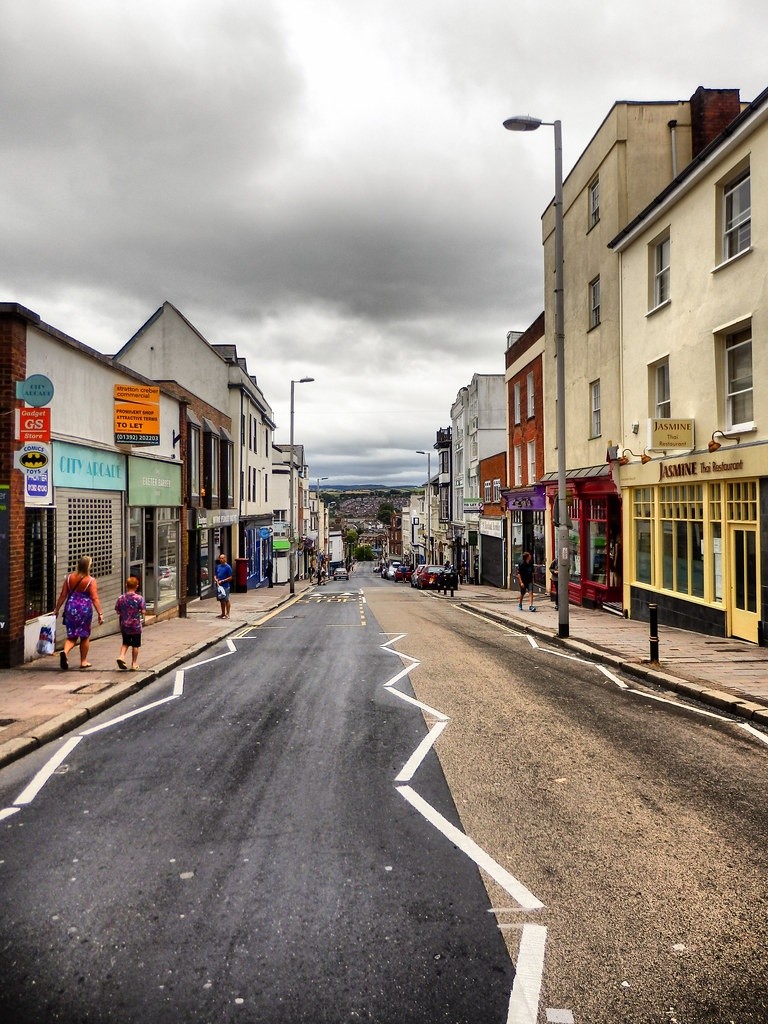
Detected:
[59, 651, 69, 670]
[117, 658, 128, 670]
[131, 665, 140, 670]
[79, 662, 92, 668]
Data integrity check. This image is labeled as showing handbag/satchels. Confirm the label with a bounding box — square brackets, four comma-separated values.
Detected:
[322, 571, 329, 576]
[35, 614, 57, 655]
[62, 612, 67, 625]
[217, 581, 226, 598]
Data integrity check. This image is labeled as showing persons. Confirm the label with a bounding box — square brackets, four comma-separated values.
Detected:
[308, 562, 328, 586]
[517, 552, 536, 611]
[214, 554, 233, 619]
[402, 564, 407, 583]
[444, 561, 465, 585]
[549, 558, 559, 610]
[53, 557, 104, 669]
[115, 576, 146, 671]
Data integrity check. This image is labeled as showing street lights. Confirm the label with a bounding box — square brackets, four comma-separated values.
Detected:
[416, 451, 431, 565]
[317, 477, 329, 585]
[290, 375, 315, 593]
[502, 115, 569, 637]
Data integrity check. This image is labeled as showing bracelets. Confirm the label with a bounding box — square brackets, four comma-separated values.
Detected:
[99, 614, 103, 615]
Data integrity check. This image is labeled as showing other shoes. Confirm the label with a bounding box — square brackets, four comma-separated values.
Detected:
[555, 605, 559, 608]
[518, 604, 522, 610]
[528, 606, 536, 611]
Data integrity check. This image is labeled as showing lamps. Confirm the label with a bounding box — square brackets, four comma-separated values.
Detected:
[708, 430, 741, 453]
[618, 448, 641, 466]
[501, 497, 531, 511]
[641, 446, 667, 465]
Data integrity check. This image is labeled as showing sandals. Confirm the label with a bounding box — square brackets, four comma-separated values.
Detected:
[222, 614, 230, 619]
[216, 615, 225, 618]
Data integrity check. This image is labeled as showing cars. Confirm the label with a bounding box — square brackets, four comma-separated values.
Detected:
[334, 568, 349, 581]
[159, 566, 177, 591]
[411, 564, 450, 591]
[372, 562, 413, 582]
